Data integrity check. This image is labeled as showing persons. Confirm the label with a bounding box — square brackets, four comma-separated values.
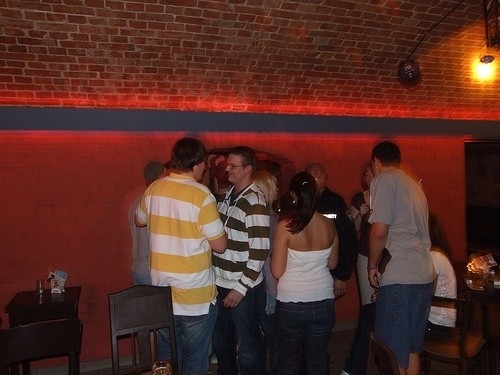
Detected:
[209, 147, 283, 375]
[428, 213, 457, 341]
[135, 138, 227, 375]
[367, 141, 437, 375]
[306, 163, 358, 301]
[270, 172, 339, 375]
[341, 161, 381, 375]
[127, 161, 172, 375]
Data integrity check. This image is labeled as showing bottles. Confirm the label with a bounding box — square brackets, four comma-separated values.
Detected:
[489, 271, 495, 287]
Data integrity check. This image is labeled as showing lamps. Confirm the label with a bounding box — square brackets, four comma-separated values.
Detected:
[398, 59, 421, 92]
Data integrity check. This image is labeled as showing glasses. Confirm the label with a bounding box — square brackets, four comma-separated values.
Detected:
[225, 162, 249, 169]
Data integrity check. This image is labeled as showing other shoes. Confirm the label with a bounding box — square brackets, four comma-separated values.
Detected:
[210, 355, 218, 364]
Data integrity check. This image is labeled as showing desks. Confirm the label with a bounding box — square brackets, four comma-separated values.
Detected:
[3, 286, 81, 375]
[456, 268, 500, 337]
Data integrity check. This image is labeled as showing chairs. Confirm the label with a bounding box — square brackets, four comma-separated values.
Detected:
[108, 285, 179, 375]
[368, 331, 400, 375]
[421, 296, 490, 375]
[0, 318, 83, 375]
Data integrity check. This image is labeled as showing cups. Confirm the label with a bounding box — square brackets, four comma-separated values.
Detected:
[152, 361, 170, 375]
[472, 273, 484, 289]
[36, 279, 44, 294]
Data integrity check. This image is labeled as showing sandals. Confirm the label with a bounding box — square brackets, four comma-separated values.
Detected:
[154, 360, 169, 375]
